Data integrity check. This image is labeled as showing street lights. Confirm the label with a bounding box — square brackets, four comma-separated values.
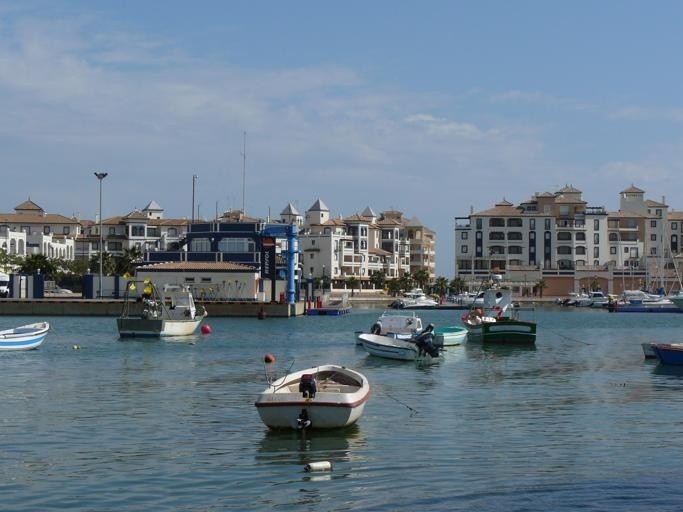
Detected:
[319, 279, 323, 290]
[487, 244, 494, 279]
[93, 172, 110, 298]
[321, 263, 326, 280]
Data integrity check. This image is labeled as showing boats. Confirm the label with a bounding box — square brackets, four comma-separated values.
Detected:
[641, 343, 658, 359]
[392, 287, 503, 310]
[114, 279, 208, 339]
[252, 426, 369, 479]
[652, 343, 682, 366]
[254, 365, 370, 431]
[0, 319, 49, 352]
[460, 272, 538, 346]
[642, 357, 682, 392]
[349, 310, 466, 363]
[466, 339, 537, 360]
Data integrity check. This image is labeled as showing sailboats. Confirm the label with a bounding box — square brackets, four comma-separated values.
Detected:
[554, 219, 682, 314]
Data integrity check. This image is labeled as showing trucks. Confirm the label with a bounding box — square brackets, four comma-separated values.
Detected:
[0, 275, 9, 298]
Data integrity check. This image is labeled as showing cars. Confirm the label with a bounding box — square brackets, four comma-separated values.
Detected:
[46, 285, 72, 294]
